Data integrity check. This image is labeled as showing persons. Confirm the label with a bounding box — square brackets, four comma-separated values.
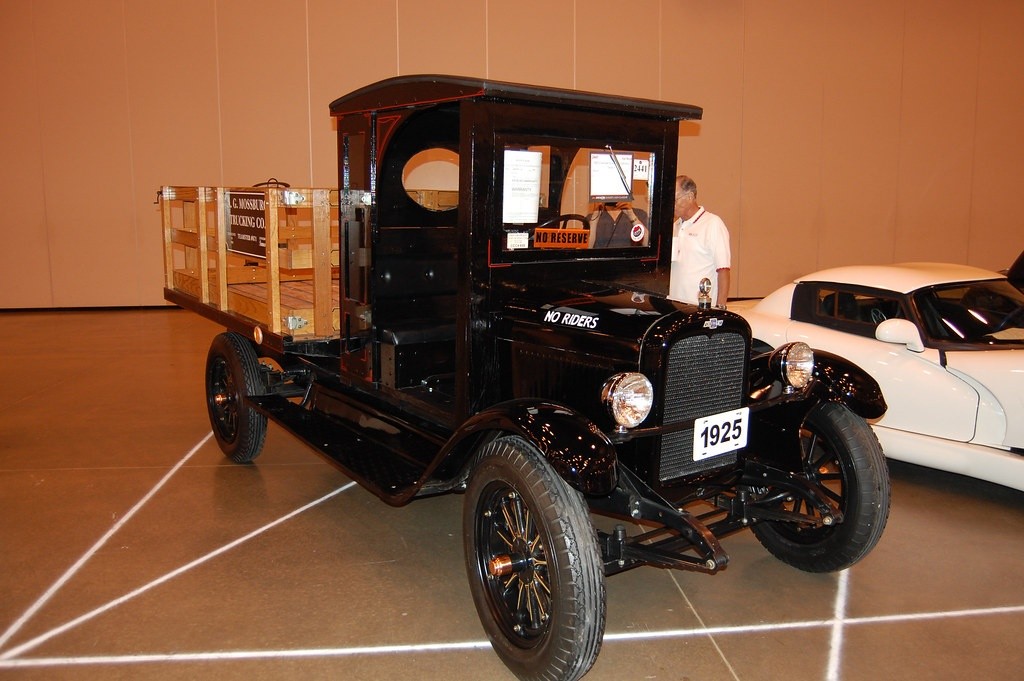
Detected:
[666, 175, 730, 310]
[586, 201, 649, 249]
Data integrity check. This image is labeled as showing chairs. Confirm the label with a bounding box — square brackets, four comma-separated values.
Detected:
[825, 293, 970, 335]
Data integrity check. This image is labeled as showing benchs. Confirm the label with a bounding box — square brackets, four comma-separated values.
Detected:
[374, 226, 463, 346]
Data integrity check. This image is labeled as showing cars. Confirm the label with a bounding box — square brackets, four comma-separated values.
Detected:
[726, 261, 1024, 492]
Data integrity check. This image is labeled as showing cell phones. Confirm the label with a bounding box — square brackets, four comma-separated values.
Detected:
[604, 202, 618, 207]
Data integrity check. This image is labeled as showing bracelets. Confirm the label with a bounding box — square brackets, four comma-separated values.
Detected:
[631, 218, 637, 224]
[716, 304, 727, 310]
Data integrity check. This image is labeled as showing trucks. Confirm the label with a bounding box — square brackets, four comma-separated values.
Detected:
[159, 74, 892, 681]
